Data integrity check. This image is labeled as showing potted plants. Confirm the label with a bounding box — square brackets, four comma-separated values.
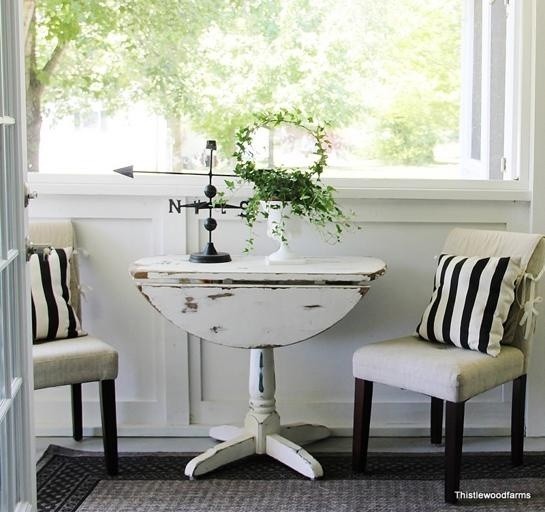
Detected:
[211, 113, 356, 264]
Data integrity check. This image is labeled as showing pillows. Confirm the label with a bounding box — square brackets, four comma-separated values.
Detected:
[416, 248, 523, 357]
[26, 242, 89, 344]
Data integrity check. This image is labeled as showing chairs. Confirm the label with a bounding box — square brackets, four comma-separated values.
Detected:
[352, 227, 545, 504]
[24, 219, 121, 480]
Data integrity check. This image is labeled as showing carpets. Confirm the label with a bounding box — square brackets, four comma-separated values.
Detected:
[35, 444, 545, 511]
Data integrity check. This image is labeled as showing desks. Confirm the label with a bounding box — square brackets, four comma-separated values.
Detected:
[129, 254, 388, 482]
